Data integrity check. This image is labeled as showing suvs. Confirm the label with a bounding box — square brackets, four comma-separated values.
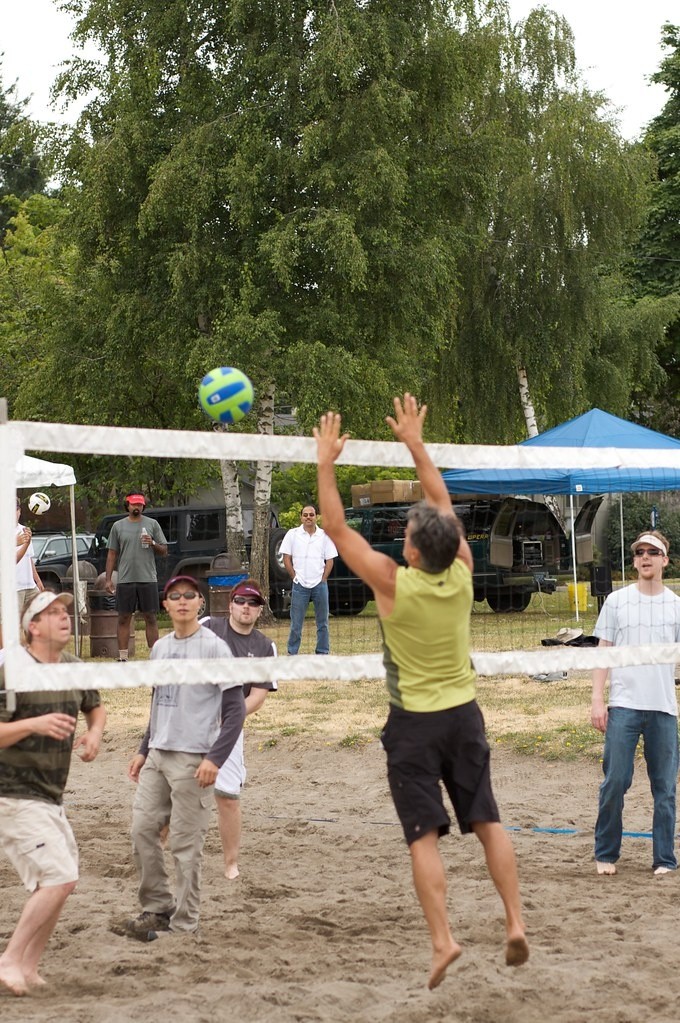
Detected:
[308, 496, 605, 618]
[33, 503, 294, 618]
[31, 531, 107, 566]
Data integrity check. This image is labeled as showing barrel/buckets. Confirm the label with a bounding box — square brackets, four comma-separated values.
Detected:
[209, 576, 249, 618]
[568, 583, 588, 612]
[86, 589, 136, 659]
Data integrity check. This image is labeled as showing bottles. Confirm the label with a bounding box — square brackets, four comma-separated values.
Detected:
[141, 528, 149, 549]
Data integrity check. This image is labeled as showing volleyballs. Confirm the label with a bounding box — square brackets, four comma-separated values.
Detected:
[199, 365, 254, 425]
[28, 492, 52, 515]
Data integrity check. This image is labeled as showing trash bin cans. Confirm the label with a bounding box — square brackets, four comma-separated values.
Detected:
[205, 552, 250, 617]
[84, 570, 136, 659]
[61, 561, 98, 637]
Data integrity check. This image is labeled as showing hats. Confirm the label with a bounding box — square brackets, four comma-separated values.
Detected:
[22, 591, 73, 633]
[164, 576, 200, 595]
[125, 494, 145, 506]
[631, 535, 667, 557]
[557, 628, 583, 643]
[231, 585, 266, 605]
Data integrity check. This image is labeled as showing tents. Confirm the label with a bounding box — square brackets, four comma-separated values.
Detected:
[0, 455, 81, 656]
[441, 408, 680, 622]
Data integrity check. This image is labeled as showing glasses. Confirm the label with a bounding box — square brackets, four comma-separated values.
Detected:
[633, 549, 661, 557]
[167, 592, 199, 600]
[232, 597, 261, 607]
[303, 513, 315, 517]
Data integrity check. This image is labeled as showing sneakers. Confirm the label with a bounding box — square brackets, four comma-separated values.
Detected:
[127, 912, 172, 941]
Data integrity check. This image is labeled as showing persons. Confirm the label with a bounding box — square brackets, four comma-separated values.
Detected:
[0, 497, 45, 650]
[591, 531, 680, 875]
[278, 505, 338, 655]
[128, 575, 279, 933]
[106, 491, 166, 661]
[312, 392, 530, 991]
[0, 588, 108, 996]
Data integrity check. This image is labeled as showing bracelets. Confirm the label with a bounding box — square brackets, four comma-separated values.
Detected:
[152, 540, 155, 545]
